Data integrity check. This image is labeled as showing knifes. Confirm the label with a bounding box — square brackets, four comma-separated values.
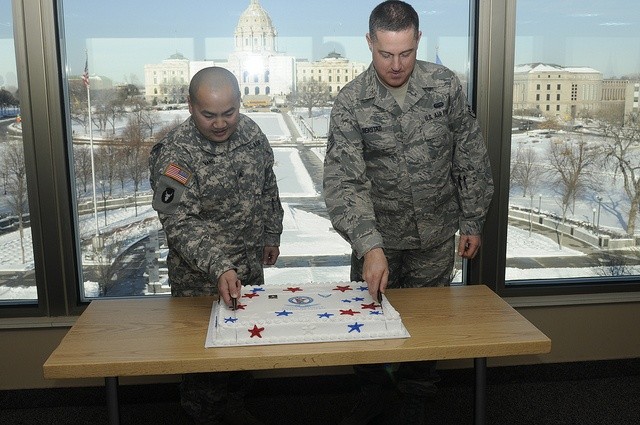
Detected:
[377, 289, 387, 331]
[232, 298, 237, 342]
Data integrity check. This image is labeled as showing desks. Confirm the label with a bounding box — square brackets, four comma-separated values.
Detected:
[43, 284, 551, 425]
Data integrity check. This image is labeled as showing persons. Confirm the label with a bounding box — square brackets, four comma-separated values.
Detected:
[322, 0, 495, 424]
[148, 67, 284, 425]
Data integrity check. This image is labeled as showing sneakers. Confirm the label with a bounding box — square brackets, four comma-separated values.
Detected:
[398, 394, 427, 425]
[339, 388, 386, 425]
[223, 397, 259, 425]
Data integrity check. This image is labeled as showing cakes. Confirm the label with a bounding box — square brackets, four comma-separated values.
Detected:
[216, 281, 403, 343]
